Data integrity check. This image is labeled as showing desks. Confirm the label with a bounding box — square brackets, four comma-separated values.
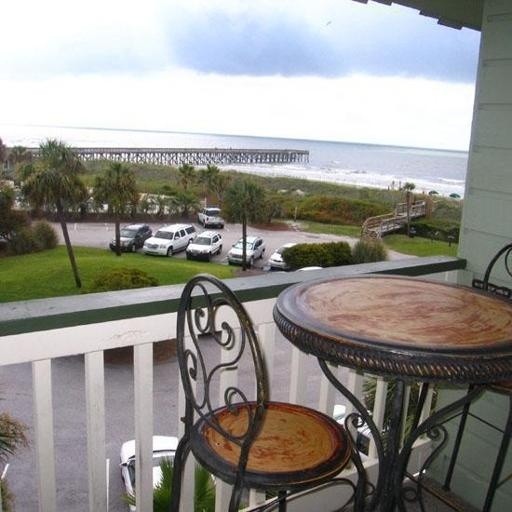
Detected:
[274, 274, 512, 512]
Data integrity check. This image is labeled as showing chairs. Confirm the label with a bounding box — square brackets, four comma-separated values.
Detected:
[169, 273, 366, 512]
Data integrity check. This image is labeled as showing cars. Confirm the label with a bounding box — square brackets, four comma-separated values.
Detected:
[268, 241, 300, 271]
[119, 434, 216, 512]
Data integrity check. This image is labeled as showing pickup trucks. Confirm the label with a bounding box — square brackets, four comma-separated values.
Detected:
[197, 207, 224, 230]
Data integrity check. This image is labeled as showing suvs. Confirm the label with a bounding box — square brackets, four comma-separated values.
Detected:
[226, 234, 266, 268]
[112, 223, 223, 263]
